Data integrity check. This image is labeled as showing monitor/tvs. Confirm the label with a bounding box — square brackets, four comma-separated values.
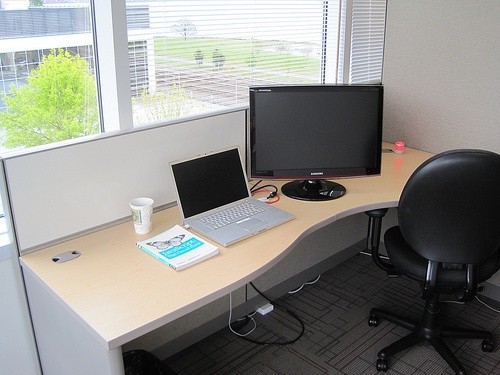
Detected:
[249, 82, 385, 202]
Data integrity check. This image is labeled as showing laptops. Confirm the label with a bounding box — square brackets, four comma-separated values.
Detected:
[169, 146, 296, 248]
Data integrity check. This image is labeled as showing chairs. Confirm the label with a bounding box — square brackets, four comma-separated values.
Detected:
[369, 149, 500, 375]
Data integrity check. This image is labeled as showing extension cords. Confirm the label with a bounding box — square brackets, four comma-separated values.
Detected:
[258, 303, 274, 316]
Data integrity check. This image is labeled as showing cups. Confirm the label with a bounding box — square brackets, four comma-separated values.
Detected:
[129, 197, 154, 235]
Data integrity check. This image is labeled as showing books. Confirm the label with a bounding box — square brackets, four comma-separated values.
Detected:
[134, 224, 219, 273]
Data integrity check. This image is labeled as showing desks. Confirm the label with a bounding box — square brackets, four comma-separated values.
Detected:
[0, 101, 440, 375]
